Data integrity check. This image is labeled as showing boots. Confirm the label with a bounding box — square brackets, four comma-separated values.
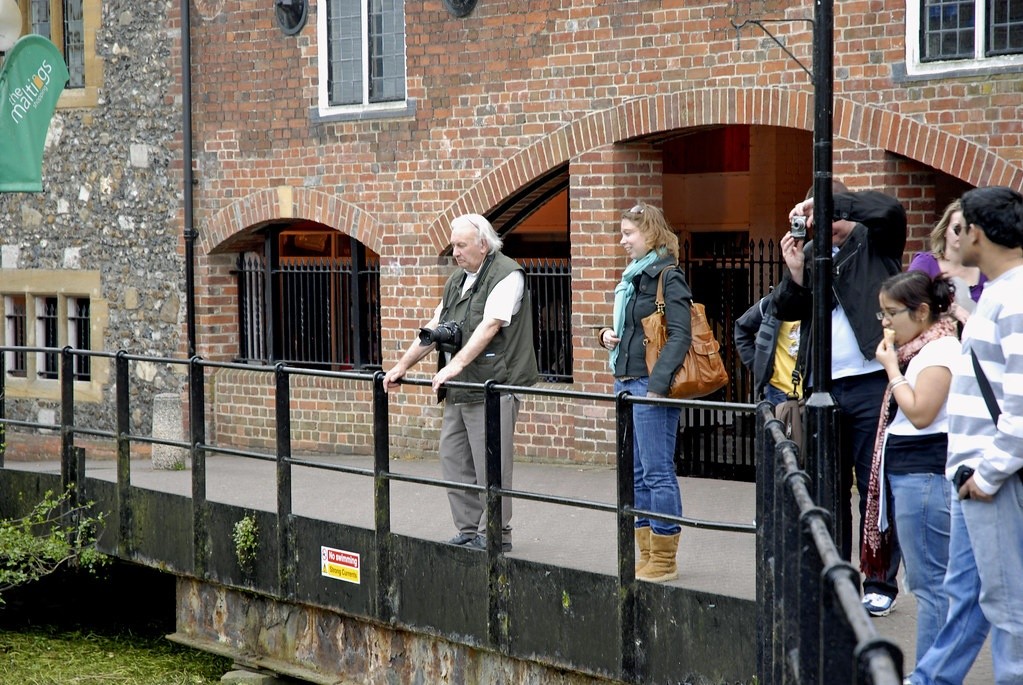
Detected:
[636, 527, 681, 582]
[634, 527, 650, 572]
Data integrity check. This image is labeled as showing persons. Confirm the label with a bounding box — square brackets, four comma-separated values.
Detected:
[382, 213, 539, 550]
[734, 281, 807, 406]
[904, 185, 1023, 685]
[907, 197, 988, 313]
[598, 203, 693, 582]
[861, 270, 973, 677]
[769, 179, 907, 617]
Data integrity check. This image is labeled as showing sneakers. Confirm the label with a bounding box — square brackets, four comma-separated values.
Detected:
[861, 593, 897, 618]
[460, 535, 511, 553]
[447, 533, 468, 544]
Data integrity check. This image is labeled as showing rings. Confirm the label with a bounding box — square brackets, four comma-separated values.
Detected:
[607, 344, 611, 347]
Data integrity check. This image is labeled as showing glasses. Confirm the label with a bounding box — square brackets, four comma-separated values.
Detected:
[876, 308, 909, 319]
[949, 222, 961, 235]
[630, 205, 644, 214]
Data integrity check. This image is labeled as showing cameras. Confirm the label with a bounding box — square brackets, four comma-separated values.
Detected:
[418, 321, 462, 354]
[791, 216, 806, 238]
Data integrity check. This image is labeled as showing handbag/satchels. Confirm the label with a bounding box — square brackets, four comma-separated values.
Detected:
[777, 396, 806, 467]
[640, 267, 729, 399]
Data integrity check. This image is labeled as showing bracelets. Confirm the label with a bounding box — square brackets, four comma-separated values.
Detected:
[888, 376, 908, 391]
